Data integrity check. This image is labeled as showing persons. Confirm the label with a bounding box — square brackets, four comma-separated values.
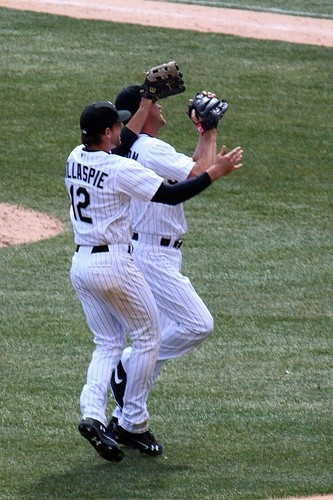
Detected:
[109, 85, 217, 448]
[63, 60, 244, 462]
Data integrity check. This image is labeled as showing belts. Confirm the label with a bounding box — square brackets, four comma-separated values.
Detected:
[131, 232, 183, 250]
[75, 244, 134, 254]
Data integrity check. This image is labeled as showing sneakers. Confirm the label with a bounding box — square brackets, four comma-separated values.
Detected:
[110, 360, 126, 413]
[77, 417, 125, 463]
[112, 423, 163, 456]
[106, 417, 118, 434]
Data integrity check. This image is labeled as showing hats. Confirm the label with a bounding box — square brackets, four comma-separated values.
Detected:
[114, 83, 142, 126]
[79, 102, 131, 134]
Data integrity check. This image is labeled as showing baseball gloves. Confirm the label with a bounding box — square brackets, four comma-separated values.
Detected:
[185, 93, 227, 132]
